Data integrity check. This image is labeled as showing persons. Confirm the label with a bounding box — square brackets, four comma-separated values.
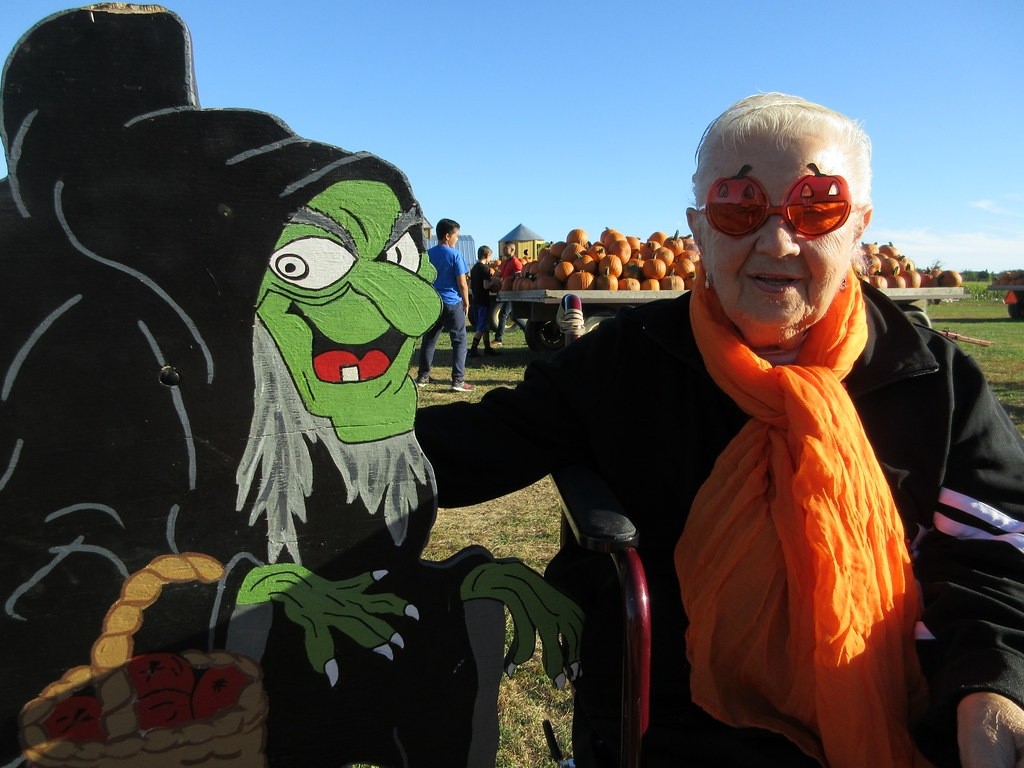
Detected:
[0, 1, 586, 768]
[417, 214, 536, 393]
[412, 91, 1024, 768]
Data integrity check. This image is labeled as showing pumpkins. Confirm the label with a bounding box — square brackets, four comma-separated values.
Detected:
[851, 242, 965, 290]
[499, 227, 701, 294]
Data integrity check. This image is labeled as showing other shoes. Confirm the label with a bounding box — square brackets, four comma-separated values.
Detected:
[489, 340, 502, 347]
[484, 349, 500, 356]
[467, 352, 480, 357]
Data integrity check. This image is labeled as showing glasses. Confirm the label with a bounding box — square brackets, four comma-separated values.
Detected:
[697, 162, 870, 240]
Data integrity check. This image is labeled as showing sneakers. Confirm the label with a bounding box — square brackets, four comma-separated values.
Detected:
[451, 380, 476, 392]
[416, 375, 433, 387]
